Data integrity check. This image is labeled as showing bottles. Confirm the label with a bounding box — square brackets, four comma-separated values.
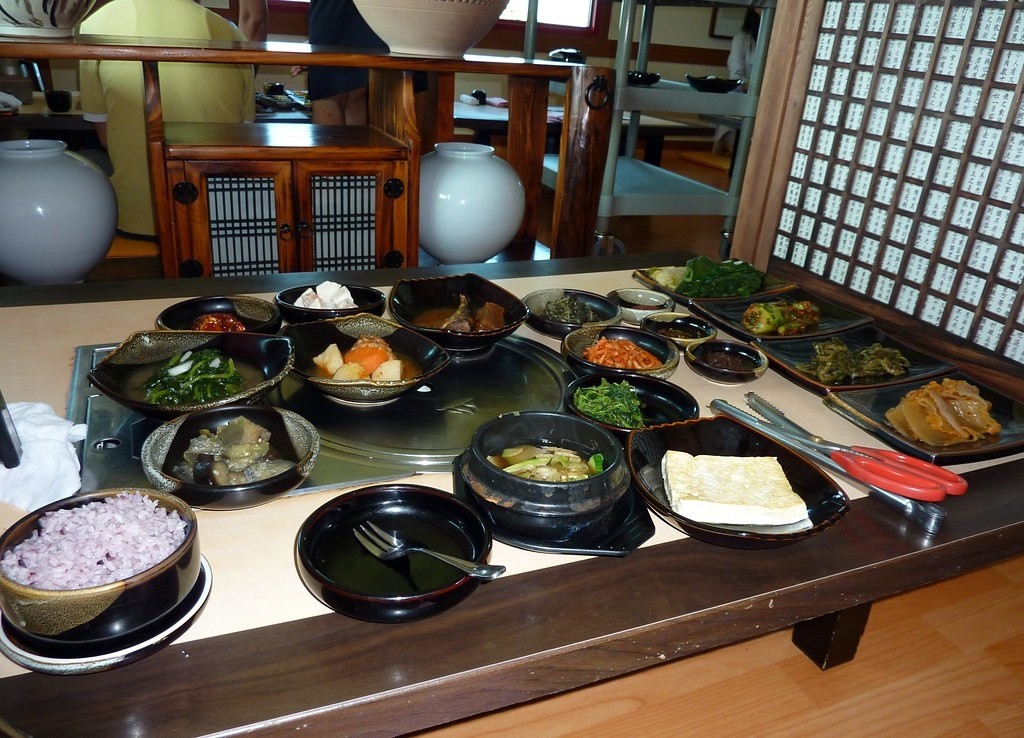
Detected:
[0, 140, 117, 284]
[418, 142, 525, 263]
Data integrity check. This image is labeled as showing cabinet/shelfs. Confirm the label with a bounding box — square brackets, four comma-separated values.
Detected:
[0, 37, 614, 276]
[520, 0, 776, 259]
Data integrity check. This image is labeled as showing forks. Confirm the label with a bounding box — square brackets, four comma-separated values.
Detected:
[352, 521, 506, 579]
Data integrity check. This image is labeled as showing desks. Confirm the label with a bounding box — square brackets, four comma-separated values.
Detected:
[254, 101, 687, 168]
[0, 260, 1024, 738]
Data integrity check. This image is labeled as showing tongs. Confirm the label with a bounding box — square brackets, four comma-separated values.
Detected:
[709, 390, 947, 536]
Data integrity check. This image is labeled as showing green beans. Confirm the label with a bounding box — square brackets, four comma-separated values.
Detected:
[540, 293, 599, 322]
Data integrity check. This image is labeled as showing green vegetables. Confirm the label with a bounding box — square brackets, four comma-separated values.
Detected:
[676, 256, 765, 298]
[796, 337, 909, 381]
[143, 349, 249, 404]
[573, 378, 646, 429]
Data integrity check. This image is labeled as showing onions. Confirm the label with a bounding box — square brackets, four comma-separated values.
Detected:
[1, 488, 187, 591]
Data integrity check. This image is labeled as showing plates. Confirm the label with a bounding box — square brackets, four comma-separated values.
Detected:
[749, 323, 960, 397]
[825, 372, 1024, 465]
[632, 257, 798, 305]
[687, 287, 873, 342]
[0, 553, 213, 675]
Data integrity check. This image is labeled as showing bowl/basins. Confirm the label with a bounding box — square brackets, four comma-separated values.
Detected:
[686, 74, 743, 94]
[141, 406, 321, 510]
[628, 70, 661, 87]
[471, 89, 487, 105]
[563, 372, 700, 447]
[560, 326, 680, 380]
[606, 287, 675, 324]
[684, 338, 768, 385]
[641, 312, 718, 351]
[523, 287, 623, 340]
[0, 488, 201, 644]
[263, 81, 285, 96]
[352, 0, 510, 58]
[452, 410, 655, 555]
[0, 0, 97, 37]
[293, 483, 493, 623]
[387, 272, 531, 352]
[274, 284, 387, 324]
[278, 312, 453, 408]
[627, 415, 851, 549]
[86, 331, 295, 421]
[153, 296, 279, 333]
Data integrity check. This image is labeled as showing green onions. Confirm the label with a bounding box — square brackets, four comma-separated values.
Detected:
[742, 298, 821, 334]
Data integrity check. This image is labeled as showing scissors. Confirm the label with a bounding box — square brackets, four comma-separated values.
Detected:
[758, 418, 968, 503]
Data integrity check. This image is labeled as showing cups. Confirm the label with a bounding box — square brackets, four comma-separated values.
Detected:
[44, 90, 72, 113]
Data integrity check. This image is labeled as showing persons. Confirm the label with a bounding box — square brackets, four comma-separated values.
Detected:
[78, 0, 268, 240]
[291, 0, 389, 126]
[715, 9, 763, 154]
